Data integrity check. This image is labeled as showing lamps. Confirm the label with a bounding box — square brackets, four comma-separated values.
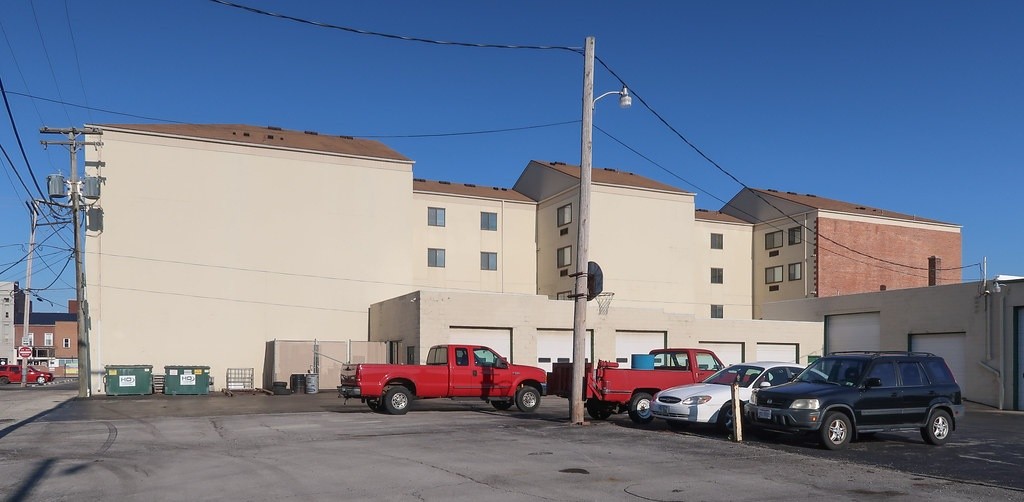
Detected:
[994, 283, 1002, 293]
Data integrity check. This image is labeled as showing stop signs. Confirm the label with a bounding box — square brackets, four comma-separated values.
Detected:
[19, 347, 33, 360]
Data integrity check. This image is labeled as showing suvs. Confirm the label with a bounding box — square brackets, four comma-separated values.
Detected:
[0, 364, 55, 385]
[743, 350, 965, 451]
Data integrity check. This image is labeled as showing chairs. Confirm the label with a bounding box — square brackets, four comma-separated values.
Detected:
[745, 373, 761, 387]
[840, 368, 859, 384]
[462, 352, 483, 366]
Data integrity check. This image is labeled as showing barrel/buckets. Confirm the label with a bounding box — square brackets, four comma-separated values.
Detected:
[631, 354, 654, 370]
[306, 373, 319, 394]
[290, 374, 306, 394]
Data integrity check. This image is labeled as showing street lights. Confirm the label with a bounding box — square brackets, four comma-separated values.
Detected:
[567, 86, 637, 424]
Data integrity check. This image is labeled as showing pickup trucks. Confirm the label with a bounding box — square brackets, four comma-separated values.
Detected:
[337, 344, 548, 415]
[547, 348, 766, 426]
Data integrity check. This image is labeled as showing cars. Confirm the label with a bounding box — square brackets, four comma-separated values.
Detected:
[648, 362, 829, 435]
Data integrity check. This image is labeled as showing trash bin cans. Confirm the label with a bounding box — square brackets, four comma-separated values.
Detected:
[164, 365, 211, 395]
[104, 364, 153, 395]
